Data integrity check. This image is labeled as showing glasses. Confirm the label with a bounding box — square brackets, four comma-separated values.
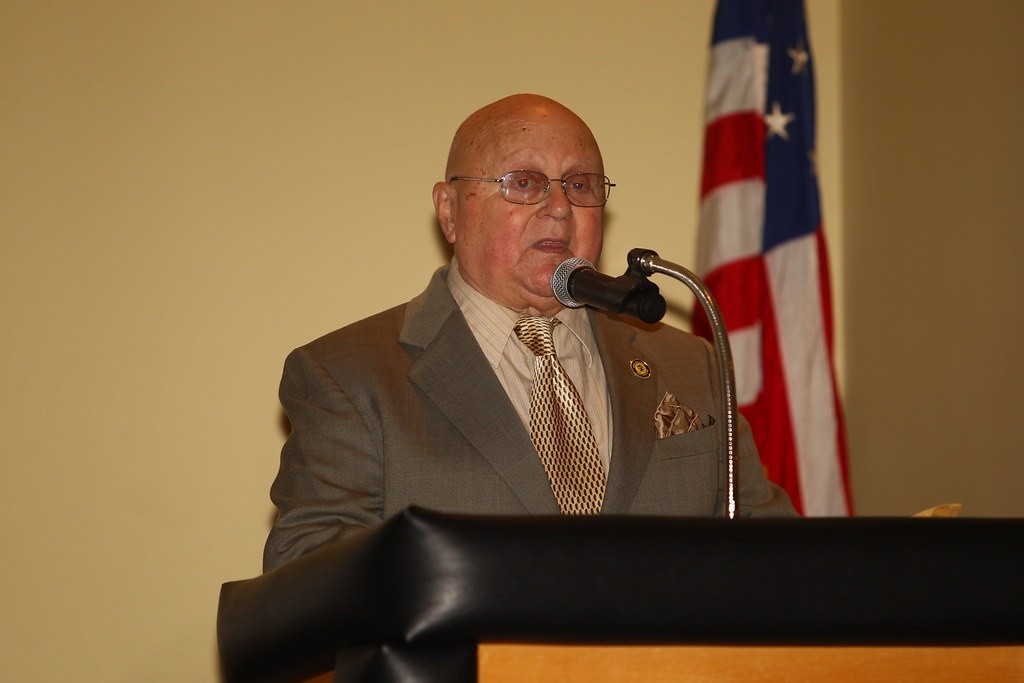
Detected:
[448, 171, 617, 209]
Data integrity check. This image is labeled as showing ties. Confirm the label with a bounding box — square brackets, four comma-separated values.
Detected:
[516, 316, 609, 516]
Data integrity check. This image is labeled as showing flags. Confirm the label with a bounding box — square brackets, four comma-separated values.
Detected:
[691, 0, 858, 519]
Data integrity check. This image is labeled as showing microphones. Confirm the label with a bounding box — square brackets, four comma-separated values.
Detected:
[550, 257, 666, 323]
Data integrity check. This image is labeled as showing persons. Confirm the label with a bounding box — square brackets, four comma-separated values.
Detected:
[261, 91, 803, 579]
